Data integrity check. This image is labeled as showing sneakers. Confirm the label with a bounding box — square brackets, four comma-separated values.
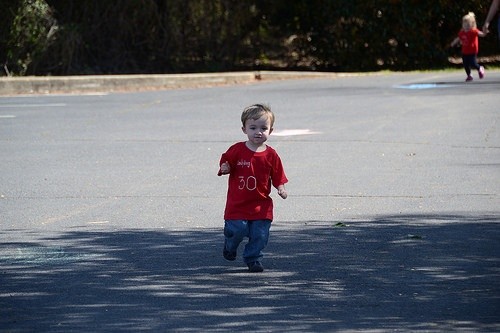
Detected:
[223, 246, 237, 261]
[246, 261, 263, 272]
[466, 75, 473, 82]
[478, 66, 484, 78]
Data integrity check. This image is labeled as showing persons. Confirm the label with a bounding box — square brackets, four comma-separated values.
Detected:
[217, 103, 288, 271]
[451, 0, 500, 81]
